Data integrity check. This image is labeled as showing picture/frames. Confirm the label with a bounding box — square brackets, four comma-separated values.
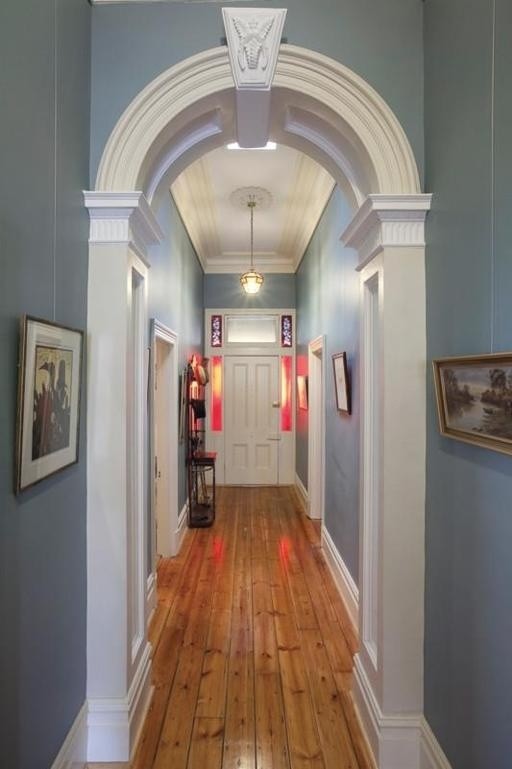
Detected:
[332, 351, 351, 415]
[296, 373, 308, 410]
[14, 312, 84, 498]
[431, 351, 512, 456]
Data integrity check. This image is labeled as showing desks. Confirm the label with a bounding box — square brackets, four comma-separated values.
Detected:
[188, 451, 217, 528]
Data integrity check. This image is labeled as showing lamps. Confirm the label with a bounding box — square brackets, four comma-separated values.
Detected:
[239, 202, 263, 295]
